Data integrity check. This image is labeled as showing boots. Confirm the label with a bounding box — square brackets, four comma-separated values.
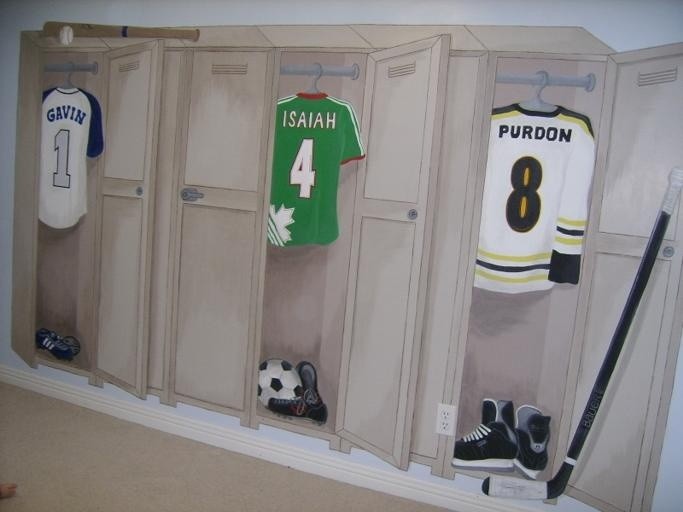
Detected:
[511, 403, 551, 472]
[448, 399, 518, 461]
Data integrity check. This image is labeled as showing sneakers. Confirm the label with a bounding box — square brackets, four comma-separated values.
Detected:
[35, 324, 82, 365]
[293, 361, 323, 407]
[263, 393, 329, 428]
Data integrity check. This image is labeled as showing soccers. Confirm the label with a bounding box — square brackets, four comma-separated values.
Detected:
[258, 358, 303, 409]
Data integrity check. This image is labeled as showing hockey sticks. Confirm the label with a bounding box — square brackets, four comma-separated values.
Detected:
[482, 163, 682, 499]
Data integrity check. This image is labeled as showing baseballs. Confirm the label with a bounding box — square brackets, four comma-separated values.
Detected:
[43, 22, 200, 44]
[59, 26, 72, 46]
